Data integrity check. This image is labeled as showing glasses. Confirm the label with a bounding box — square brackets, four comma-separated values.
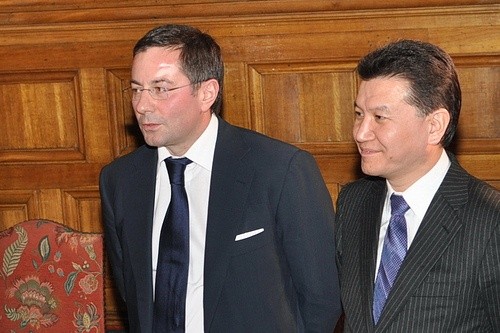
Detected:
[122, 81, 199, 99]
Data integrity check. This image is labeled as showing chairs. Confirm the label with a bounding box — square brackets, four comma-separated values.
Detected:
[0, 218, 107, 333]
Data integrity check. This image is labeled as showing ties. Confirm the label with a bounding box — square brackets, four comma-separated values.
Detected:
[153, 156, 194, 333]
[372, 194, 411, 326]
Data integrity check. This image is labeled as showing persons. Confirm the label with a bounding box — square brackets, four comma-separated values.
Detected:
[329, 39, 500, 333]
[100, 25, 343, 333]
